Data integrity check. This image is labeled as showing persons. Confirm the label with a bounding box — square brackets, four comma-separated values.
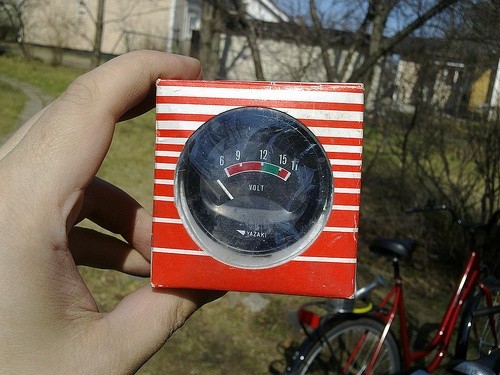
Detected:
[0, 49, 232, 374]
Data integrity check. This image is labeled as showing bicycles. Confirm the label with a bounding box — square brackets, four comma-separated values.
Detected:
[274, 197, 500, 375]
